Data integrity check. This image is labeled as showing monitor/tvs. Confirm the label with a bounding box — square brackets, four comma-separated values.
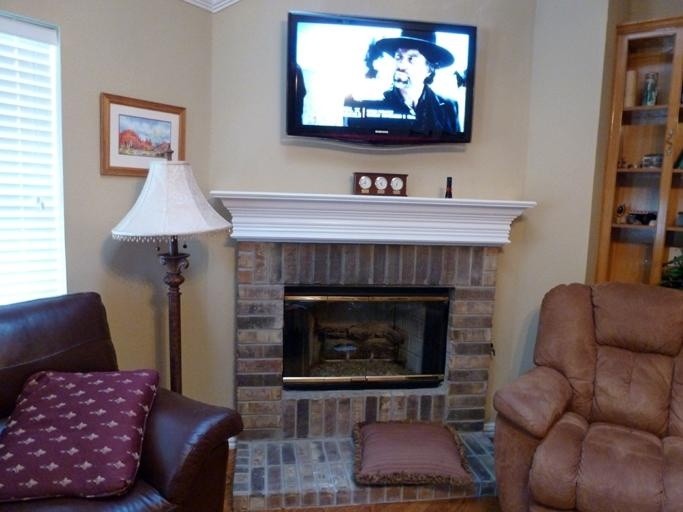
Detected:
[278, 11, 478, 154]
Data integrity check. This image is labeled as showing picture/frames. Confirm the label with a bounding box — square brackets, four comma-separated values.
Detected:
[100, 92, 186, 177]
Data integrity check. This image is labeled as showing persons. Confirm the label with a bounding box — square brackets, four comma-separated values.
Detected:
[341, 31, 460, 133]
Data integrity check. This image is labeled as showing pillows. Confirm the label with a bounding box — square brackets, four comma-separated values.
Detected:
[0, 368, 159, 504]
[351, 419, 476, 488]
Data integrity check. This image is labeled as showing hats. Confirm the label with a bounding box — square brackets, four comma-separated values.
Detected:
[376, 30, 455, 70]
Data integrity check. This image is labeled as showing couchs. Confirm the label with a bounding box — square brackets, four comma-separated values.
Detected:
[0, 292, 244, 512]
[493, 281, 683, 512]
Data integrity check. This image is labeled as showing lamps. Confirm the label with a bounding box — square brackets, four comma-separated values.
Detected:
[111, 160, 232, 395]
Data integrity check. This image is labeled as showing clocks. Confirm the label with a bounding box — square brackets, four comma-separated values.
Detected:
[353, 172, 408, 196]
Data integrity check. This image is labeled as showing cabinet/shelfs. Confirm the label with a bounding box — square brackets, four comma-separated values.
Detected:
[595, 16, 683, 285]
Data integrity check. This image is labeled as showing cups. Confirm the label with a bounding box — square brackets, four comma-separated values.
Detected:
[642, 71, 659, 106]
